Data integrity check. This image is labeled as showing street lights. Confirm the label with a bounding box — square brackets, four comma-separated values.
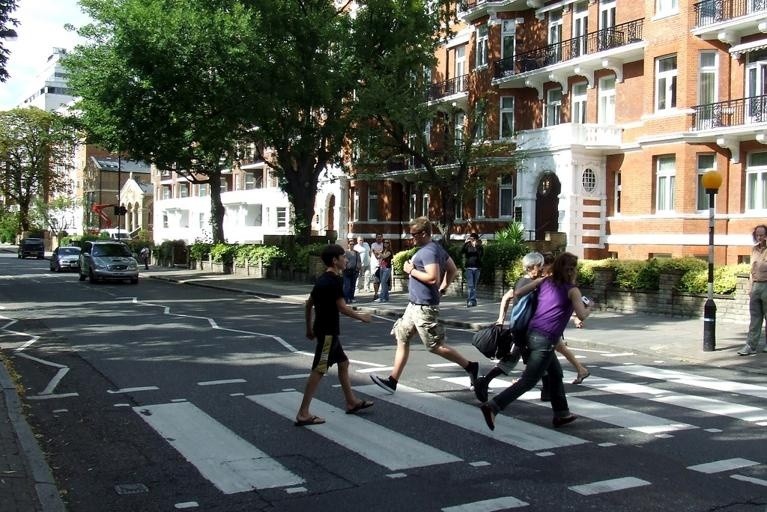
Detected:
[699, 166, 725, 353]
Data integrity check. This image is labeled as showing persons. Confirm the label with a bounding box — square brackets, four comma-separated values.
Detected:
[339, 240, 361, 303]
[468, 248, 594, 431]
[369, 214, 478, 393]
[292, 242, 376, 427]
[733, 223, 767, 356]
[352, 236, 373, 293]
[372, 239, 394, 302]
[368, 233, 385, 301]
[139, 244, 150, 271]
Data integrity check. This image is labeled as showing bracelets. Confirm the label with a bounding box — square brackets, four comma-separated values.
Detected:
[406, 267, 416, 279]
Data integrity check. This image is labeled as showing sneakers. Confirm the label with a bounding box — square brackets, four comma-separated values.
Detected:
[347, 298, 357, 304]
[551, 413, 578, 427]
[737, 344, 757, 356]
[464, 361, 478, 386]
[373, 295, 388, 302]
[480, 402, 496, 432]
[368, 373, 399, 395]
[466, 300, 476, 307]
[473, 377, 488, 402]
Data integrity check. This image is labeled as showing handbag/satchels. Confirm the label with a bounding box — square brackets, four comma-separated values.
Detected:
[343, 268, 356, 278]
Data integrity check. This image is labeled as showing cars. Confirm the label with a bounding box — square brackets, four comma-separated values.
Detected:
[48, 246, 82, 273]
[78, 239, 139, 285]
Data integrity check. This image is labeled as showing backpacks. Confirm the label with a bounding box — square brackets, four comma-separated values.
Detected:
[510, 289, 538, 346]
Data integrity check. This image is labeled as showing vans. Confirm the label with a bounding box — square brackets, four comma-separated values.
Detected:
[97, 227, 132, 242]
[16, 236, 46, 260]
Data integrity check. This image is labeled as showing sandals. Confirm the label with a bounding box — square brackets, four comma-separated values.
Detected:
[572, 366, 590, 385]
[293, 414, 326, 427]
[344, 399, 376, 414]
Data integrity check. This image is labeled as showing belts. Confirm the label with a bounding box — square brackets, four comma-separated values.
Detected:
[409, 299, 439, 306]
[752, 280, 767, 283]
